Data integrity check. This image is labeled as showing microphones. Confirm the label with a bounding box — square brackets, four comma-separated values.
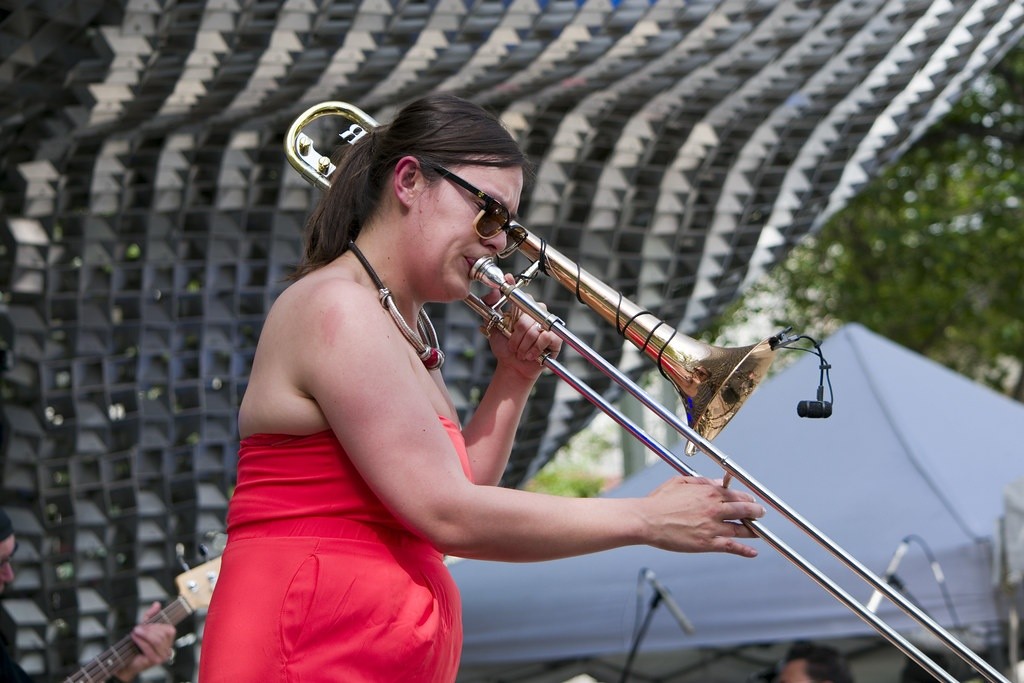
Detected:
[866, 538, 910, 614]
[797, 400, 832, 418]
[641, 567, 695, 636]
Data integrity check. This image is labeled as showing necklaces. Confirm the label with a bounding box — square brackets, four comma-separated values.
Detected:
[350, 240, 445, 371]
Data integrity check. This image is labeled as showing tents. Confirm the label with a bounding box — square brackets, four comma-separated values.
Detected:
[445, 326, 1024, 683]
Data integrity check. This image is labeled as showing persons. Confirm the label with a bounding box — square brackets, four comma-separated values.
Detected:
[200, 95, 765, 683]
[0, 508, 177, 683]
[779, 640, 852, 683]
[901, 650, 947, 683]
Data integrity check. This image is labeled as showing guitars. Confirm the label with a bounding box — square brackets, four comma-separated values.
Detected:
[63, 543, 222, 683]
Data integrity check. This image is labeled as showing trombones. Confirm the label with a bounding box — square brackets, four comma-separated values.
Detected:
[284, 100, 1024, 682]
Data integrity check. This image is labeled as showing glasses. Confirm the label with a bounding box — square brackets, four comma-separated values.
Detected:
[425, 158, 527, 261]
